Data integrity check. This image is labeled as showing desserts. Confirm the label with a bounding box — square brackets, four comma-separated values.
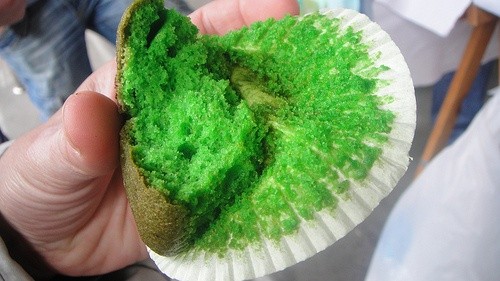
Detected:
[115, 1, 393, 255]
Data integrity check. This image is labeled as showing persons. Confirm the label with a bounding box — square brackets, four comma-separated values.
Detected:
[0, 1, 301, 281]
[0, 0, 143, 125]
[426, 58, 500, 165]
